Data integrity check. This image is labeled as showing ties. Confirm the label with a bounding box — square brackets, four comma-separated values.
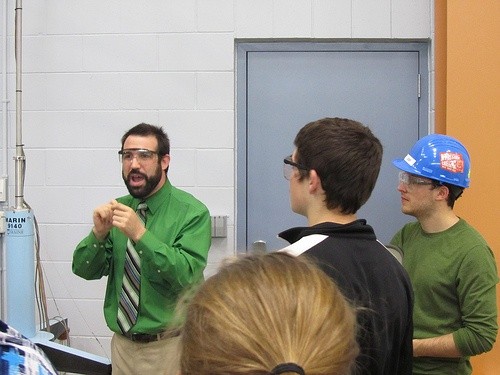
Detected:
[116, 203, 149, 334]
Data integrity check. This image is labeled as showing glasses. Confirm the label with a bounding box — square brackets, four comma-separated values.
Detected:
[398, 171, 433, 185]
[283, 154, 309, 178]
[119, 148, 159, 162]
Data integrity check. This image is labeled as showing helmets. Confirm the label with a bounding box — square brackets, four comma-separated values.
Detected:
[392, 134, 471, 188]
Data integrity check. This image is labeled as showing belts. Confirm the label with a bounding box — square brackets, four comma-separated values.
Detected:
[122, 331, 181, 342]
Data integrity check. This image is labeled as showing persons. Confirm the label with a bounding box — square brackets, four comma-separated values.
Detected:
[384, 133, 499, 375]
[71, 122, 211, 375]
[179, 251, 360, 375]
[276, 115, 415, 375]
